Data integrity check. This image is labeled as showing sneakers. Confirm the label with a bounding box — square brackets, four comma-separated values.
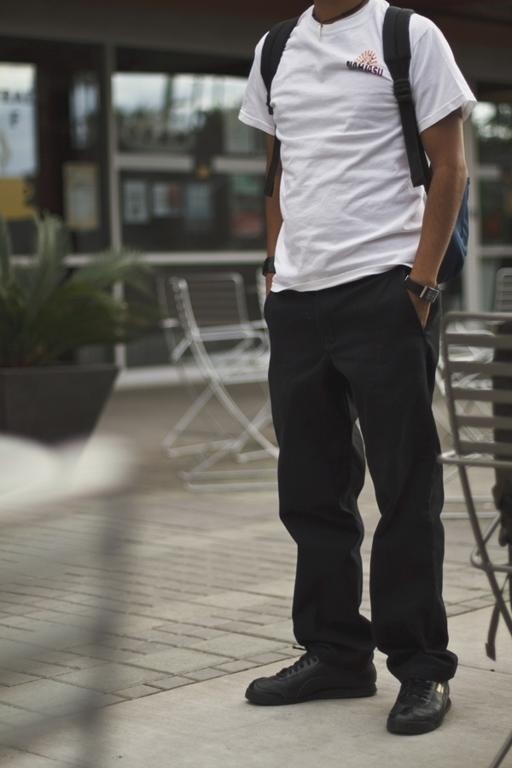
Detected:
[244, 644, 379, 708]
[385, 676, 455, 738]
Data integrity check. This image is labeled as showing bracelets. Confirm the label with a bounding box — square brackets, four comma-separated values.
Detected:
[262, 256, 278, 277]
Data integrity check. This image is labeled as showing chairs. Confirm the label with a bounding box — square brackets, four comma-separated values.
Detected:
[431, 268, 512, 659]
[153, 268, 282, 492]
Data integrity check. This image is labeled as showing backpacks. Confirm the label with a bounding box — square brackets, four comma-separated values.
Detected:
[258, 5, 472, 284]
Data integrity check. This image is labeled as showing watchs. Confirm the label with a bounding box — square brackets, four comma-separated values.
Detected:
[403, 274, 440, 306]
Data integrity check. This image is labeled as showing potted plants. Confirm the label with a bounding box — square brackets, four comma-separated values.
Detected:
[0, 213, 135, 440]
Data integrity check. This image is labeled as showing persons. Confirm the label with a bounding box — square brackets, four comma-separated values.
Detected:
[232, 0, 476, 740]
